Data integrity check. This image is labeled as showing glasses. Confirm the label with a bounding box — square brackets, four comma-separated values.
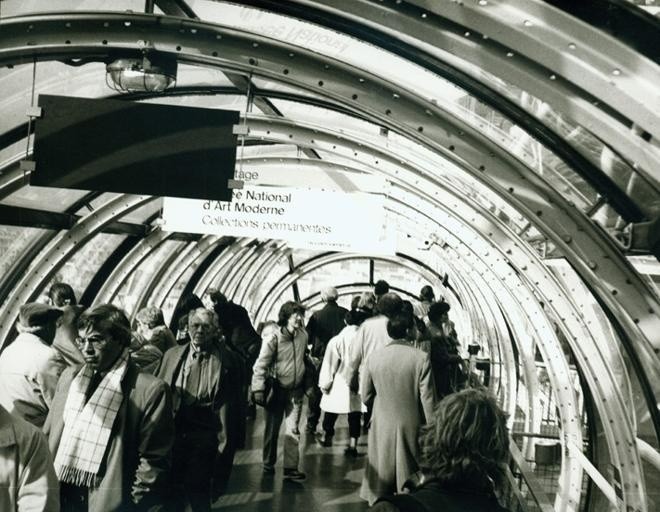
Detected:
[74, 337, 104, 351]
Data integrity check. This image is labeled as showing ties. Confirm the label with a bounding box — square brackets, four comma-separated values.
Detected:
[184, 353, 203, 406]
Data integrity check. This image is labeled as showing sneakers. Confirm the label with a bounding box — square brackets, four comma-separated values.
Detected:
[344, 447, 358, 458]
[317, 437, 332, 448]
[262, 466, 275, 476]
[282, 468, 306, 480]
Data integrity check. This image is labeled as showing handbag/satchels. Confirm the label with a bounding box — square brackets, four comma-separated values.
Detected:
[251, 334, 281, 411]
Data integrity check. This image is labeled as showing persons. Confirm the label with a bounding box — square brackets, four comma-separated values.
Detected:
[318, 311, 364, 457]
[179, 294, 206, 330]
[0, 404, 60, 512]
[349, 293, 404, 397]
[402, 299, 427, 341]
[413, 284, 437, 317]
[49, 283, 85, 365]
[374, 280, 390, 303]
[366, 389, 513, 512]
[1, 302, 66, 428]
[157, 308, 240, 512]
[357, 290, 376, 324]
[251, 302, 309, 480]
[359, 313, 438, 507]
[42, 304, 173, 512]
[211, 289, 262, 420]
[305, 287, 350, 433]
[347, 296, 361, 326]
[135, 306, 179, 353]
[426, 301, 463, 401]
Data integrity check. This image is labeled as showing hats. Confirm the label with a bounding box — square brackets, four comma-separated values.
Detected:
[16, 302, 64, 333]
